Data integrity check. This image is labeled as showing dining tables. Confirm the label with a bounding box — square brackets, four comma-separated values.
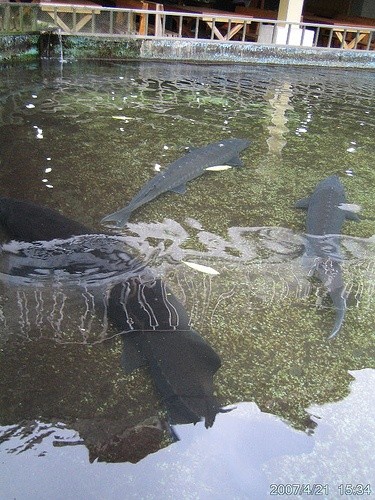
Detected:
[302, 14, 374, 50]
[39, 1, 104, 34]
[163, 4, 254, 40]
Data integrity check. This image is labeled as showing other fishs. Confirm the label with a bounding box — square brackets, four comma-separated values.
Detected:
[292, 175, 361, 340]
[0, 196, 222, 432]
[99, 140, 255, 225]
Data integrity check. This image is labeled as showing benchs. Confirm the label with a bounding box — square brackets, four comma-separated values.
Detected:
[227, 6, 279, 42]
[323, 15, 375, 50]
[149, 2, 182, 37]
[98, 1, 147, 36]
